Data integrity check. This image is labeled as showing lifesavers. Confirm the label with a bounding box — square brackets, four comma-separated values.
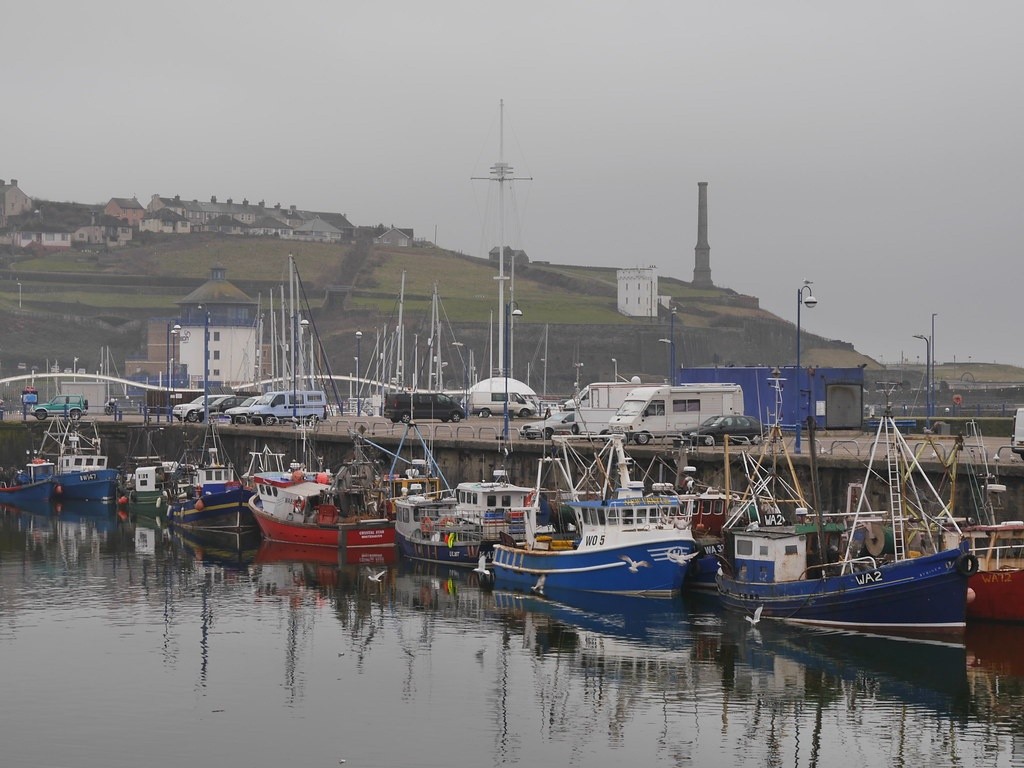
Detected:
[526, 490, 535, 506]
[292, 495, 305, 513]
[956, 553, 978, 575]
[439, 517, 455, 532]
[421, 516, 434, 534]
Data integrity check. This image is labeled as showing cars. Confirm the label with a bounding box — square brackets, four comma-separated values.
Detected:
[173, 395, 251, 423]
[681, 414, 766, 446]
[521, 410, 575, 440]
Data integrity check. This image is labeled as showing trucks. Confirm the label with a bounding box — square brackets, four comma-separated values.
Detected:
[608, 383, 745, 445]
[563, 382, 664, 411]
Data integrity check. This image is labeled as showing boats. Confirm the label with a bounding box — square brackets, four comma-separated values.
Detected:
[0, 381, 1024, 629]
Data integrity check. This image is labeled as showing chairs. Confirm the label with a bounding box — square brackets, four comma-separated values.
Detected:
[723, 421, 728, 425]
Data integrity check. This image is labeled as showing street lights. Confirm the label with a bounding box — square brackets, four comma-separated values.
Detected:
[166, 319, 182, 423]
[931, 313, 938, 416]
[504, 301, 522, 439]
[355, 325, 363, 418]
[659, 338, 675, 385]
[794, 286, 818, 453]
[913, 334, 929, 430]
[198, 302, 208, 424]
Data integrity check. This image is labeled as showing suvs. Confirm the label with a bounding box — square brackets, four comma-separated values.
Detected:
[30, 395, 88, 420]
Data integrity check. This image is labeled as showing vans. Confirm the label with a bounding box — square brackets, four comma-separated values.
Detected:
[249, 391, 326, 425]
[1010, 407, 1024, 462]
[384, 392, 466, 423]
[471, 391, 537, 418]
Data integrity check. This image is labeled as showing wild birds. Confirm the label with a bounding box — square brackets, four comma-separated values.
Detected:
[365, 566, 389, 582]
[471, 555, 490, 575]
[616, 554, 654, 574]
[664, 551, 700, 567]
[745, 606, 763, 627]
[530, 573, 545, 596]
